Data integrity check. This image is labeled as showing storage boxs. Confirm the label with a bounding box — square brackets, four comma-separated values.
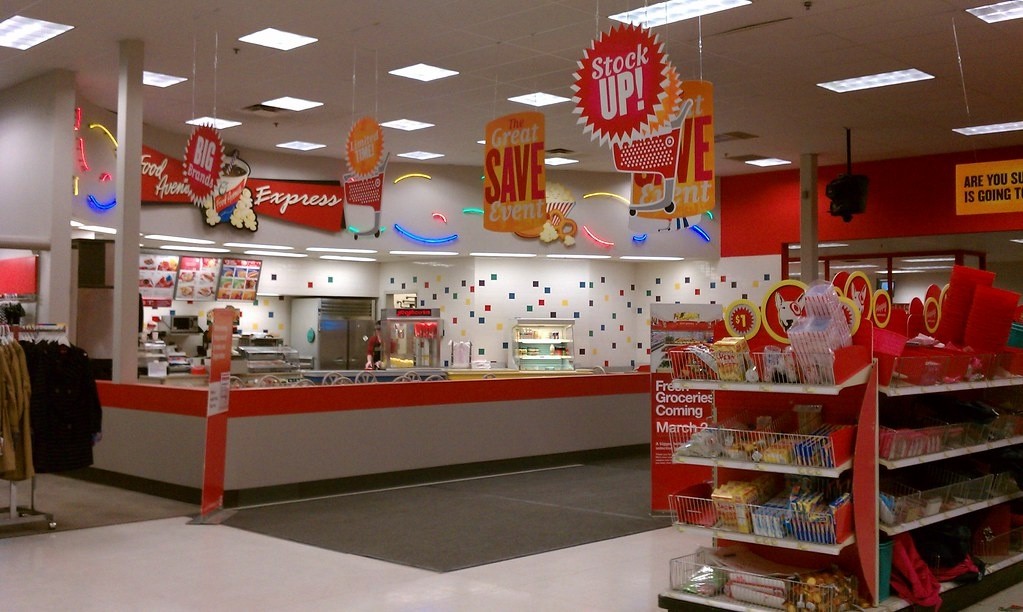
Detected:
[764, 436, 803, 464]
[715, 336, 753, 382]
[878, 402, 1003, 461]
[713, 473, 789, 539]
[880, 467, 1013, 556]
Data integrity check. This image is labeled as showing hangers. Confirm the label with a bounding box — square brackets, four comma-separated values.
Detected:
[0, 323, 15, 345]
[21, 324, 71, 346]
[0, 292, 19, 306]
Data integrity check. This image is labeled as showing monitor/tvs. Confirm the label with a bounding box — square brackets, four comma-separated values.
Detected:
[71, 239, 115, 289]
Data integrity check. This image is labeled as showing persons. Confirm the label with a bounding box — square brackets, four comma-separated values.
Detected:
[365, 320, 396, 372]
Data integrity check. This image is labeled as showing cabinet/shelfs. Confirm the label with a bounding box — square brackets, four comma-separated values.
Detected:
[650, 301, 1023, 612]
[507, 317, 576, 372]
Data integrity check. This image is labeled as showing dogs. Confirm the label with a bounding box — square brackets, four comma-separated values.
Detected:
[775, 291, 807, 334]
[851, 283, 866, 314]
